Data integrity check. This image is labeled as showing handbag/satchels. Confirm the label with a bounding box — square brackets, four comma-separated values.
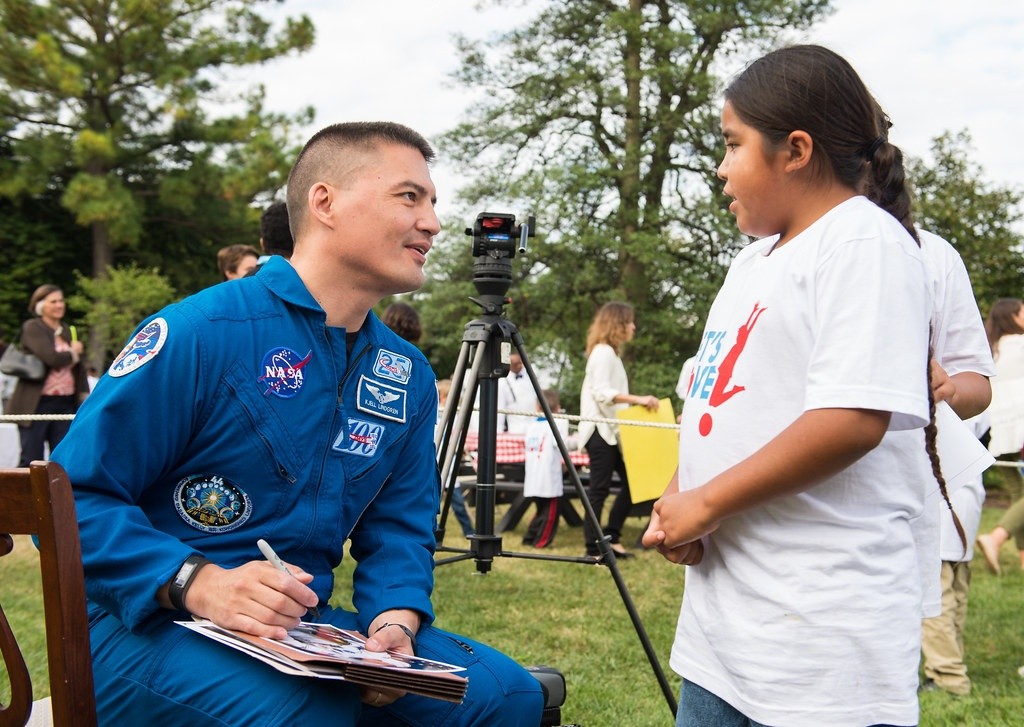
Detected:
[1, 342, 47, 426]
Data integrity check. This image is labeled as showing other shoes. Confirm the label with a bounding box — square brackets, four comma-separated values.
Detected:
[585, 554, 599, 559]
[610, 546, 636, 560]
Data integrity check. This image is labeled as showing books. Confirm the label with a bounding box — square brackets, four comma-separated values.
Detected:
[174, 615, 469, 705]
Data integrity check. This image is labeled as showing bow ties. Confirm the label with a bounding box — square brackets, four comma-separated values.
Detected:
[515, 373, 523, 378]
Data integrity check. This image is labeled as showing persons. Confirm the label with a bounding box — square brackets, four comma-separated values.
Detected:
[575, 302, 658, 561]
[5, 286, 90, 468]
[674, 351, 697, 424]
[642, 44, 1024, 727]
[496, 351, 539, 432]
[382, 303, 423, 348]
[522, 388, 569, 549]
[976, 298, 1024, 577]
[911, 228, 996, 695]
[216, 243, 259, 282]
[33, 120, 546, 727]
[432, 380, 474, 542]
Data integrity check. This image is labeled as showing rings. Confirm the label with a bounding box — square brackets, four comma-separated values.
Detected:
[369, 692, 382, 705]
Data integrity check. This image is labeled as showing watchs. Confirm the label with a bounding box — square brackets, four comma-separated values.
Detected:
[168, 554, 212, 615]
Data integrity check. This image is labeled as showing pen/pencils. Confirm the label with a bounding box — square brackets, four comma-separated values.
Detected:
[257, 538, 322, 620]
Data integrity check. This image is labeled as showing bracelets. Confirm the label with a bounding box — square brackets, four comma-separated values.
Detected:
[373, 622, 419, 657]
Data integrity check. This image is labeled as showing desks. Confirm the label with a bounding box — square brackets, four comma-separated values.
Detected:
[463, 433, 591, 533]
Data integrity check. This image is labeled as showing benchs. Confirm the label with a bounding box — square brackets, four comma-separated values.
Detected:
[461, 480, 622, 534]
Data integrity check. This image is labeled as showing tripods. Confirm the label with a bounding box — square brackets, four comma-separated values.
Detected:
[435, 276, 678, 719]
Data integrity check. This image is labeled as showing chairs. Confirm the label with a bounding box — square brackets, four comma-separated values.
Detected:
[0, 459, 96, 727]
